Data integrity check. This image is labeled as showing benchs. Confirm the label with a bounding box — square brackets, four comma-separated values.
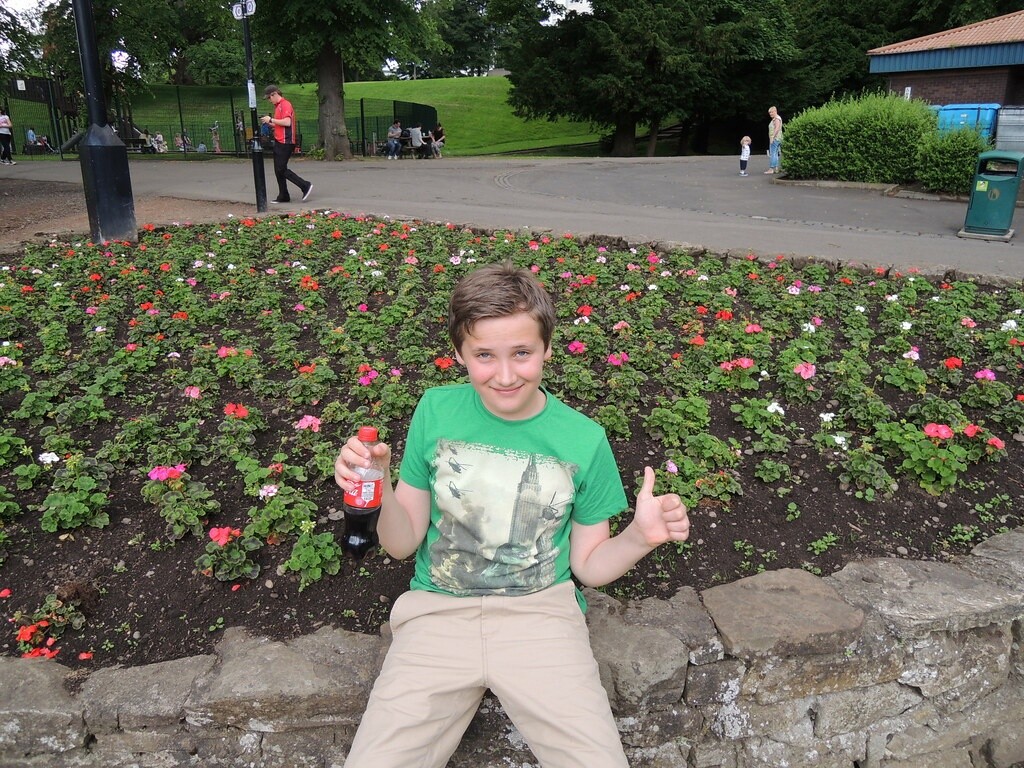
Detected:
[120, 138, 147, 154]
[404, 147, 418, 149]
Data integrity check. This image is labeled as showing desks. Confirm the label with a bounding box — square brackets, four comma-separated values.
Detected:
[400, 136, 430, 159]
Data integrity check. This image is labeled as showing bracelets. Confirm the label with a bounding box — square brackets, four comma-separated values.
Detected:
[269, 117, 272, 123]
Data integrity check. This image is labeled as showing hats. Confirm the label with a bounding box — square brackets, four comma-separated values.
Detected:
[263, 85, 278, 100]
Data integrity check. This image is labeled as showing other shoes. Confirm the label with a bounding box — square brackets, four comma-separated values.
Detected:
[302, 184, 314, 201]
[764, 169, 774, 174]
[739, 172, 748, 177]
[775, 169, 778, 173]
[394, 155, 399, 159]
[270, 200, 278, 204]
[388, 155, 392, 160]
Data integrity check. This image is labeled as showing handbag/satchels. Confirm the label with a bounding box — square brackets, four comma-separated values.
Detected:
[401, 128, 410, 137]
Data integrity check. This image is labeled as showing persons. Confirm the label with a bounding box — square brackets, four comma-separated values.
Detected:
[334, 265, 689, 768]
[738, 136, 751, 176]
[199, 141, 207, 152]
[155, 131, 163, 144]
[410, 124, 430, 159]
[386, 119, 402, 160]
[259, 85, 312, 204]
[259, 115, 269, 136]
[764, 106, 782, 174]
[144, 125, 153, 137]
[236, 118, 243, 134]
[175, 128, 192, 150]
[27, 126, 38, 144]
[0, 106, 17, 165]
[429, 123, 445, 159]
[40, 135, 54, 152]
[209, 121, 221, 152]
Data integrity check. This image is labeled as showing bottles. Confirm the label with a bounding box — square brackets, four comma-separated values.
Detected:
[340, 426, 385, 562]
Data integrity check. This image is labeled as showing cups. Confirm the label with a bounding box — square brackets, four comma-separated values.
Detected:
[423, 133, 425, 137]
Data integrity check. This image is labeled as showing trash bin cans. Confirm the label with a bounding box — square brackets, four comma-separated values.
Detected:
[963, 150, 1024, 236]
